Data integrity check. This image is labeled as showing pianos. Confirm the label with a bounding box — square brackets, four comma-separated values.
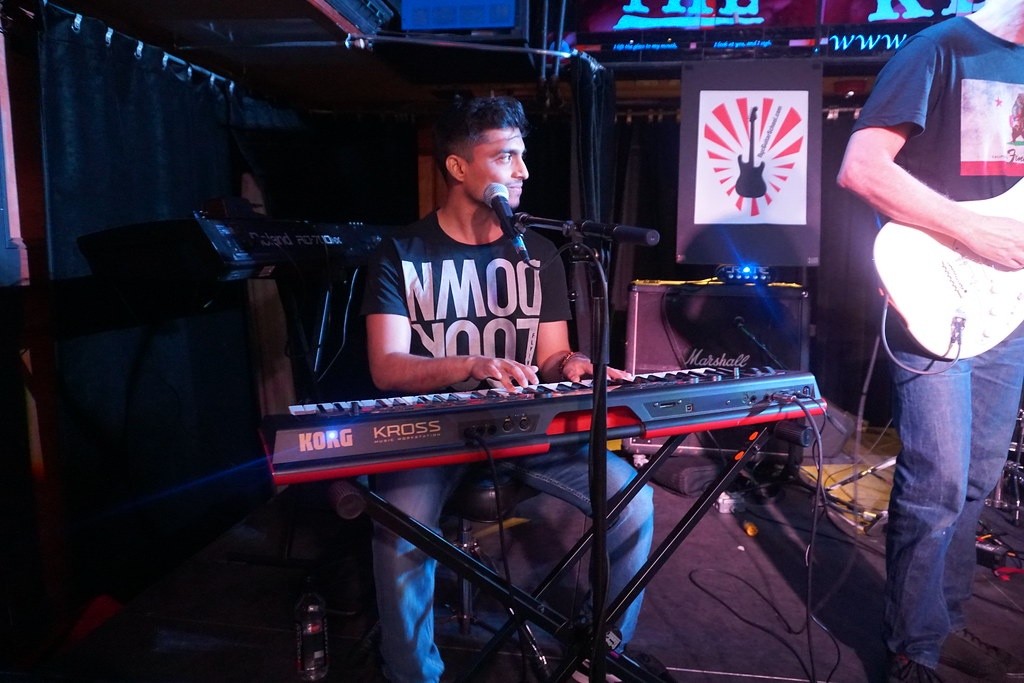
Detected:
[252, 365, 827, 487]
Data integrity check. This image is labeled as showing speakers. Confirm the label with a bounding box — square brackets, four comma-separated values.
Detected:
[624, 282, 810, 465]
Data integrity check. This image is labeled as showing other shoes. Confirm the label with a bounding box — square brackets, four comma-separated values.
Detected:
[572, 658, 622, 683]
[938, 629, 1024, 683]
[884, 641, 944, 683]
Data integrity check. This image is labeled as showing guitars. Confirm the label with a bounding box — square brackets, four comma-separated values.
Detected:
[872, 177, 1024, 364]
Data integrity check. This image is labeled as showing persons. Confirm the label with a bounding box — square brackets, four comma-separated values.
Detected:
[366, 95, 658, 683]
[833, 1, 1024, 683]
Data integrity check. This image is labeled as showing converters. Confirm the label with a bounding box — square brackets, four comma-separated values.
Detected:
[975, 538, 1007, 569]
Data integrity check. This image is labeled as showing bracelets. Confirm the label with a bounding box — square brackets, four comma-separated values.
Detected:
[558, 351, 590, 380]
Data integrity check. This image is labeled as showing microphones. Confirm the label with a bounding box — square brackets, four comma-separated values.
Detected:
[484, 182, 530, 264]
